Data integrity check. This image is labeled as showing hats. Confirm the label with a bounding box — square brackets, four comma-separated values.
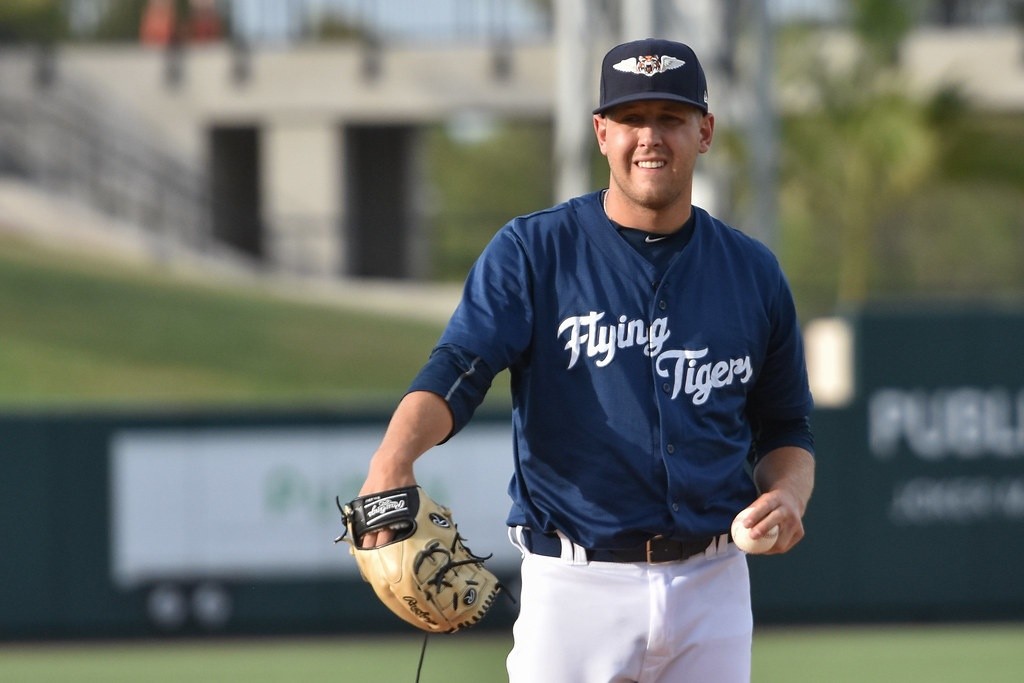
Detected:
[592, 38, 709, 115]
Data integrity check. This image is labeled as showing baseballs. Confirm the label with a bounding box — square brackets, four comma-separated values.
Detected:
[730, 508, 780, 555]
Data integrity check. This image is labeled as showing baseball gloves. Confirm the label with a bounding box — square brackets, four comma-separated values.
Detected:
[332, 486, 516, 639]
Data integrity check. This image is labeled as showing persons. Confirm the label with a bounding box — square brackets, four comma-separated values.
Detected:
[335, 38, 816, 683]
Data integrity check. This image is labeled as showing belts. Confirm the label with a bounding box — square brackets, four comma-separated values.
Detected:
[522, 524, 734, 566]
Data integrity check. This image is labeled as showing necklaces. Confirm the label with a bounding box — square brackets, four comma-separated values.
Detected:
[603, 188, 612, 220]
